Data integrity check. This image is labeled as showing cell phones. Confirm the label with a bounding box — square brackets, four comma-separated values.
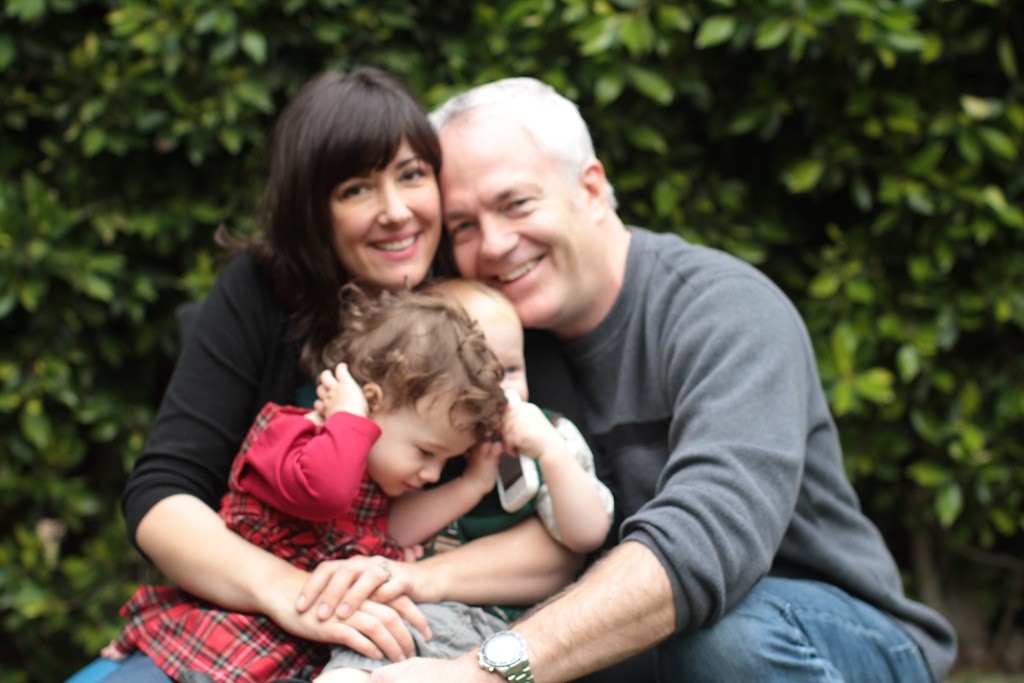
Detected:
[496, 452, 540, 513]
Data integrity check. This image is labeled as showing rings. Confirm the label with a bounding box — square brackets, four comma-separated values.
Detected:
[382, 566, 391, 581]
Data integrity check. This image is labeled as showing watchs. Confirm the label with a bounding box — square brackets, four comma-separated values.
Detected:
[478, 630, 534, 683]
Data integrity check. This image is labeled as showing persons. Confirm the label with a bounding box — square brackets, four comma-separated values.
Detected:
[373, 77, 960, 683]
[117, 67, 583, 663]
[315, 278, 615, 683]
[95, 290, 509, 683]
